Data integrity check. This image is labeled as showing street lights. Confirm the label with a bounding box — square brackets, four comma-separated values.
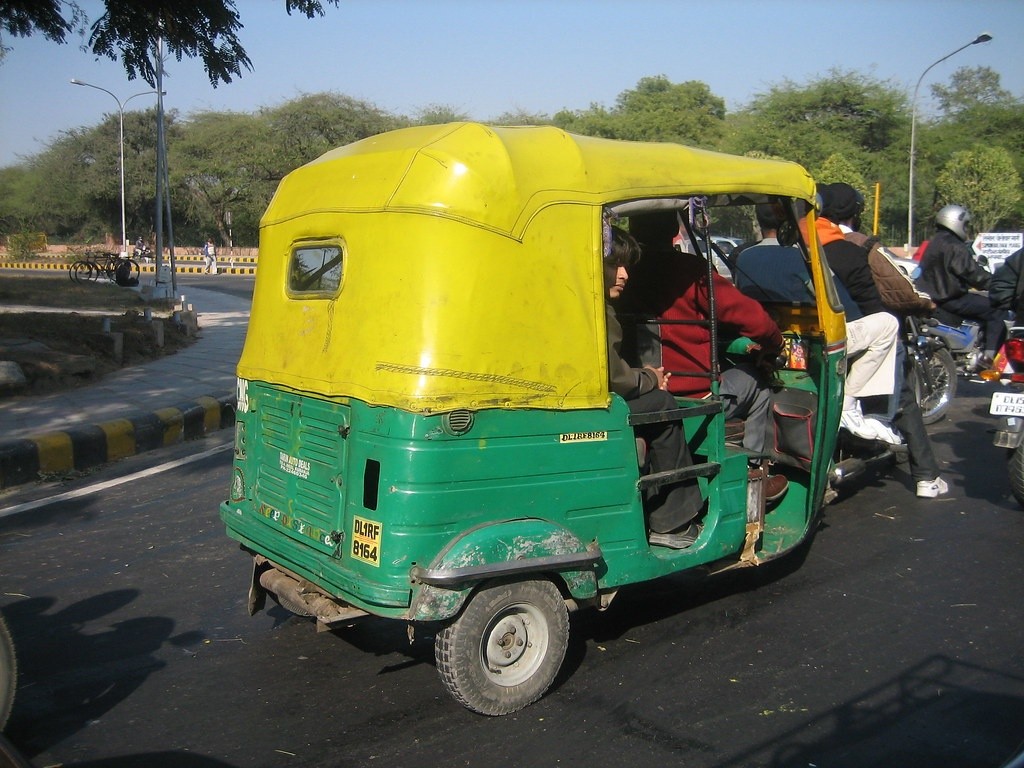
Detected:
[906, 30, 992, 261]
[69, 78, 167, 253]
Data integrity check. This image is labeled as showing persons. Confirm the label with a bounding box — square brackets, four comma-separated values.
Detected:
[989, 246, 1024, 338]
[134, 237, 147, 259]
[629, 209, 788, 501]
[605, 226, 706, 550]
[913, 203, 1013, 378]
[735, 202, 900, 440]
[204, 237, 218, 275]
[116, 260, 139, 287]
[795, 181, 949, 497]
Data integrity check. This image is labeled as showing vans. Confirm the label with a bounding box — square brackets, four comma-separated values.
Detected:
[687, 234, 746, 279]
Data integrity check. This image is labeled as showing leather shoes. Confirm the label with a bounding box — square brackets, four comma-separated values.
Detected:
[977, 356, 994, 369]
[747, 466, 789, 500]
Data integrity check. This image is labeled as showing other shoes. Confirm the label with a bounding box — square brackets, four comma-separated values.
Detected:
[649, 518, 705, 549]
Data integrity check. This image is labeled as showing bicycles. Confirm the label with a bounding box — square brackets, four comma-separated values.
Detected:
[68, 249, 141, 286]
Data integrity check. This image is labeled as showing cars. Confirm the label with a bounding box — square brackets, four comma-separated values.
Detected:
[879, 245, 922, 280]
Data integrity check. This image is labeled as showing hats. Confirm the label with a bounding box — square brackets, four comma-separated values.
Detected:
[819, 183, 865, 220]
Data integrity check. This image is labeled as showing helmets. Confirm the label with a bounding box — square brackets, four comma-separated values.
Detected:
[935, 205, 971, 242]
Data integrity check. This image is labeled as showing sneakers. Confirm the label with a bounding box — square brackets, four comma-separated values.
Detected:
[865, 418, 902, 444]
[839, 401, 877, 439]
[916, 476, 948, 498]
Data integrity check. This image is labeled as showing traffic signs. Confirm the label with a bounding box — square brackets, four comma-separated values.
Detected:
[972, 232, 1024, 261]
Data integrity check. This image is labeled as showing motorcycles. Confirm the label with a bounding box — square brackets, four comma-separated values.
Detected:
[215, 122, 850, 719]
[827, 264, 1024, 511]
